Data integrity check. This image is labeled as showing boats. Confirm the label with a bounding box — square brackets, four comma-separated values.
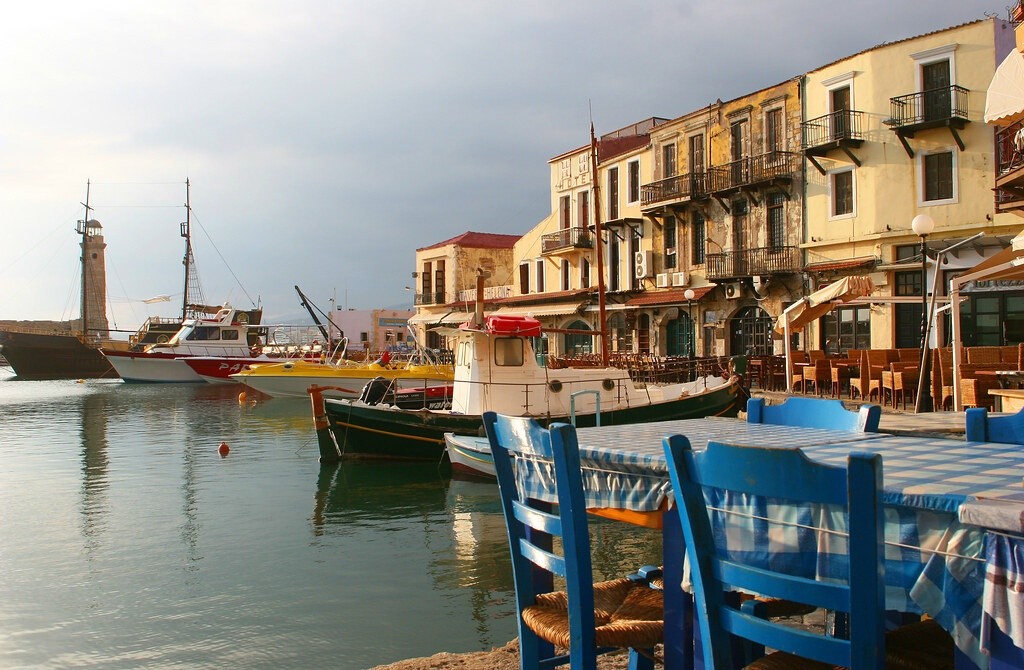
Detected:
[174, 330, 390, 384]
[98, 309, 329, 383]
[444, 432, 516, 480]
[228, 337, 455, 400]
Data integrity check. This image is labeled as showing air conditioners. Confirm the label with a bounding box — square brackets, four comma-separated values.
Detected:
[725, 282, 744, 300]
[672, 272, 689, 286]
[635, 251, 653, 279]
[656, 273, 672, 288]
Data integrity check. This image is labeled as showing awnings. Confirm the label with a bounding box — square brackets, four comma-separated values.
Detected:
[441, 312, 476, 324]
[984, 47, 1024, 124]
[407, 312, 452, 325]
[495, 301, 586, 317]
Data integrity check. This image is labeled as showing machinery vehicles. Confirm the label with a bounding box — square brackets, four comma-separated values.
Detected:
[295, 285, 350, 363]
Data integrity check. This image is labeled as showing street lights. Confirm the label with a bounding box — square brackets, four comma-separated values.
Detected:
[912, 214, 934, 413]
[404, 287, 417, 355]
[684, 290, 696, 382]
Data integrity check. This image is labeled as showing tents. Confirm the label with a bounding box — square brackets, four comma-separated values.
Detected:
[949, 229, 1024, 412]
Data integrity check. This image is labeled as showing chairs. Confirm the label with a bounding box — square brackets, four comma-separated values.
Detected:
[547, 352, 729, 384]
[482, 411, 665, 669]
[767, 343, 1024, 412]
[662, 432, 929, 670]
[637, 397, 887, 640]
[887, 406, 1024, 670]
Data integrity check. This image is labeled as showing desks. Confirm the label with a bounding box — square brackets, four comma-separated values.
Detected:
[975, 370, 1024, 411]
[958, 494, 1024, 670]
[835, 362, 859, 398]
[513, 418, 891, 670]
[681, 436, 1024, 670]
[794, 362, 815, 395]
[872, 364, 890, 406]
[749, 360, 767, 389]
[903, 366, 920, 369]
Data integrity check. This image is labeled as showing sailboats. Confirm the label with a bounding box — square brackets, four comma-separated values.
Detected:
[0, 177, 262, 379]
[322, 122, 747, 462]
